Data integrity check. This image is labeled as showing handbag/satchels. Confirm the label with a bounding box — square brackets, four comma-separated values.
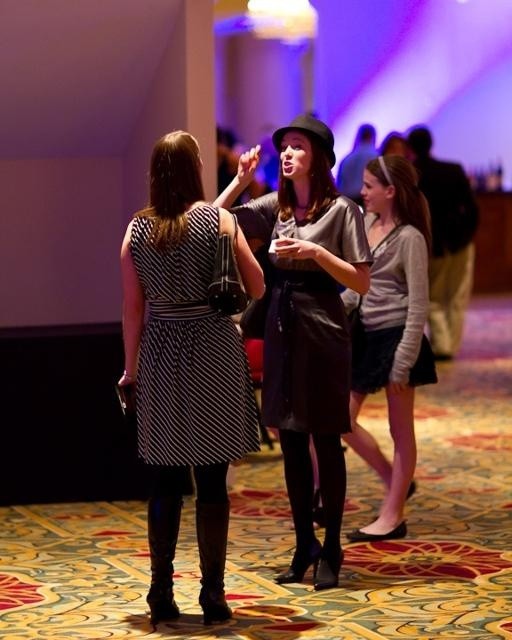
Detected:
[206, 213, 250, 316]
[348, 307, 360, 333]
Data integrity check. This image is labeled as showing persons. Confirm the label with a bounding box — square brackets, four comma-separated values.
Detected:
[212, 114, 373, 591]
[341, 156, 439, 541]
[114, 130, 268, 627]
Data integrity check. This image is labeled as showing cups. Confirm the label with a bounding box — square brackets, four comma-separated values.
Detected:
[118, 380, 142, 415]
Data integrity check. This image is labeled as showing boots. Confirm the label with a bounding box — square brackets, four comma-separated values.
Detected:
[146, 501, 182, 623]
[195, 499, 234, 625]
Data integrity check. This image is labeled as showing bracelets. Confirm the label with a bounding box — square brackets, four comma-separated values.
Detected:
[123, 368, 136, 383]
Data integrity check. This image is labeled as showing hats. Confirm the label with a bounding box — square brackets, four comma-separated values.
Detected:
[272, 115, 336, 169]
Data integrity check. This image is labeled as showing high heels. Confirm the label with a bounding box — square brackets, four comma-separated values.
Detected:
[274, 540, 322, 584]
[315, 550, 344, 591]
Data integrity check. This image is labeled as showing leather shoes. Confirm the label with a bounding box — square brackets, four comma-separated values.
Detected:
[372, 481, 415, 524]
[346, 520, 407, 542]
